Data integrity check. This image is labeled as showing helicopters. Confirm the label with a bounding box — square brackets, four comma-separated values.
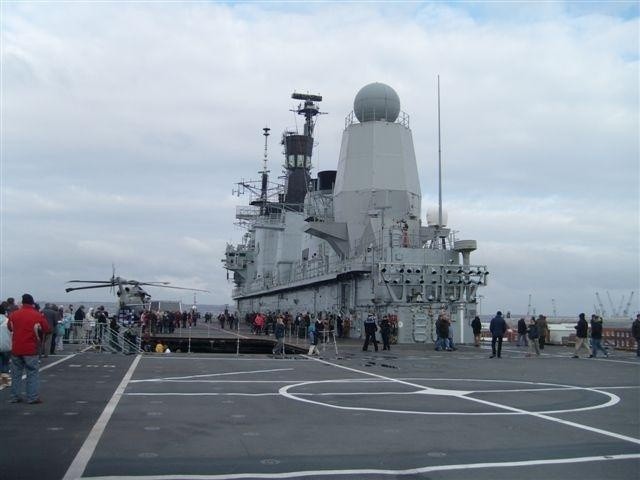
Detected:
[65, 262, 213, 316]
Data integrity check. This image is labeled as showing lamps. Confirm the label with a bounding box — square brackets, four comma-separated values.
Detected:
[379, 263, 489, 303]
[368, 242, 373, 252]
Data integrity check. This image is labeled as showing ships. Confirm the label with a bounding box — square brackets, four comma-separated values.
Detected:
[0, 69, 640, 477]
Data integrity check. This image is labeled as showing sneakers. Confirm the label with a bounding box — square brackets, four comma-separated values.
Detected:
[572, 351, 611, 361]
[361, 347, 391, 352]
[434, 346, 458, 352]
[490, 355, 503, 359]
[516, 344, 545, 357]
[9, 393, 44, 405]
